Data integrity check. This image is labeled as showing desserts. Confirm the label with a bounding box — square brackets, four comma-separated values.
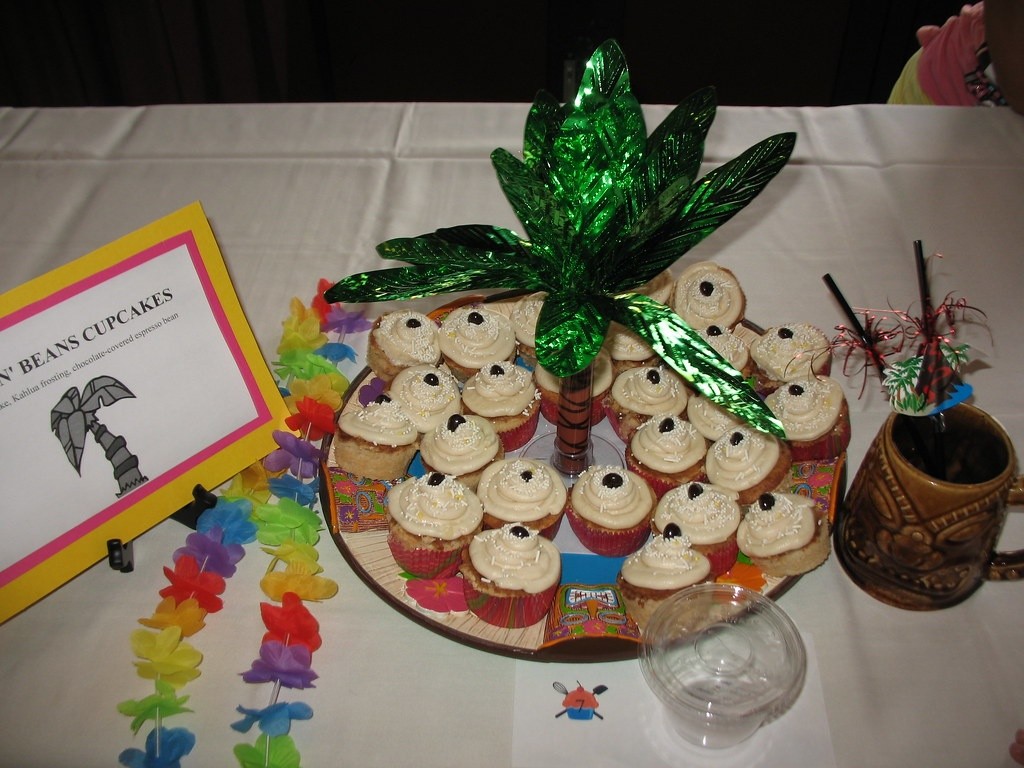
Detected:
[335, 261, 853, 630]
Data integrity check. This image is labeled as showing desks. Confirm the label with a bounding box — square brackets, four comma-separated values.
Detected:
[0, 106, 1023, 768]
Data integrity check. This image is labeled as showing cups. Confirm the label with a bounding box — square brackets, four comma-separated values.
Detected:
[833, 401, 1024, 612]
[641, 582, 807, 746]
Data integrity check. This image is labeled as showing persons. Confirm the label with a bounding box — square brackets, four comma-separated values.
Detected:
[884, 0, 1024, 116]
